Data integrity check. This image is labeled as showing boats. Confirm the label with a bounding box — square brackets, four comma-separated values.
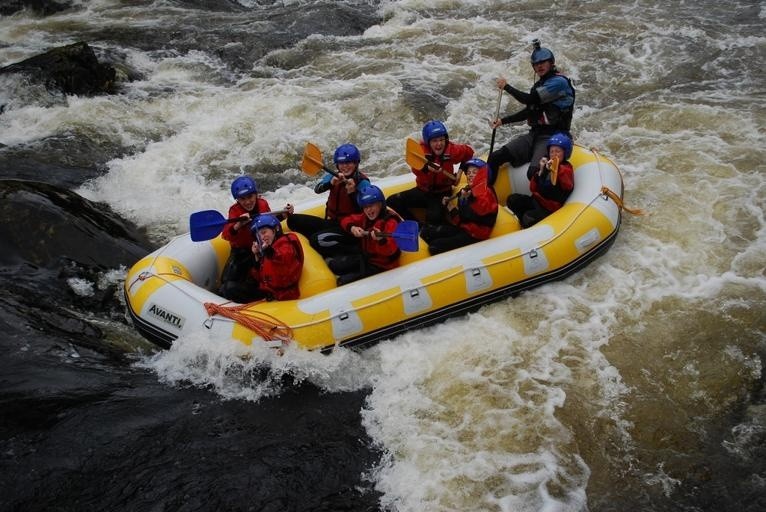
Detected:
[123, 140, 627, 364]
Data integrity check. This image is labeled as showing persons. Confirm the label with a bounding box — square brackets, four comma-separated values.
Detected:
[507, 133, 574, 229]
[386, 121, 474, 231]
[487, 48, 575, 186]
[221, 176, 294, 284]
[420, 159, 498, 255]
[224, 214, 303, 304]
[287, 144, 370, 257]
[329, 185, 401, 287]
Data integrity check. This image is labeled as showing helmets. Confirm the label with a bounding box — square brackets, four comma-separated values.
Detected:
[250, 214, 284, 239]
[230, 177, 257, 199]
[545, 133, 573, 160]
[530, 47, 556, 74]
[356, 184, 385, 207]
[421, 120, 448, 143]
[332, 143, 361, 163]
[461, 158, 492, 183]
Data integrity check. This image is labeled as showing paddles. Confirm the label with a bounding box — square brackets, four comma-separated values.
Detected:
[405, 138, 456, 180]
[545, 156, 559, 186]
[446, 164, 488, 203]
[360, 221, 419, 251]
[301, 142, 349, 184]
[190, 204, 293, 243]
